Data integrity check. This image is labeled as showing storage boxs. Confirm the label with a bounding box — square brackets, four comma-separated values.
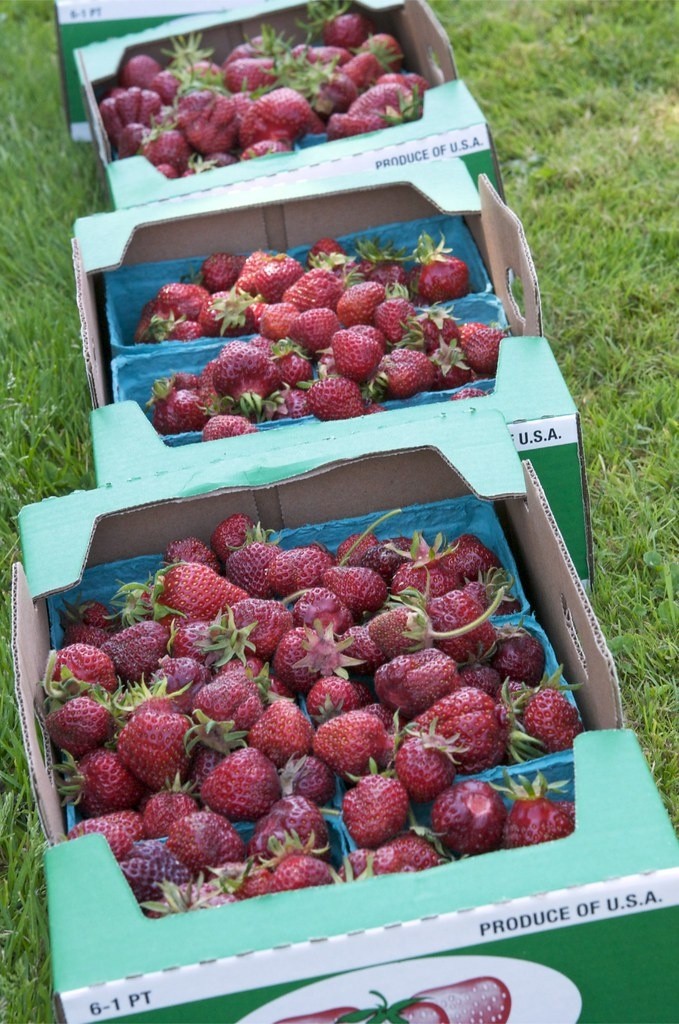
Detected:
[10, 0, 679, 1024]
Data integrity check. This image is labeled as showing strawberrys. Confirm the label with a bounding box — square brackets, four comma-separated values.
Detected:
[40, 514, 582, 916]
[95, 11, 430, 179]
[138, 233, 509, 443]
